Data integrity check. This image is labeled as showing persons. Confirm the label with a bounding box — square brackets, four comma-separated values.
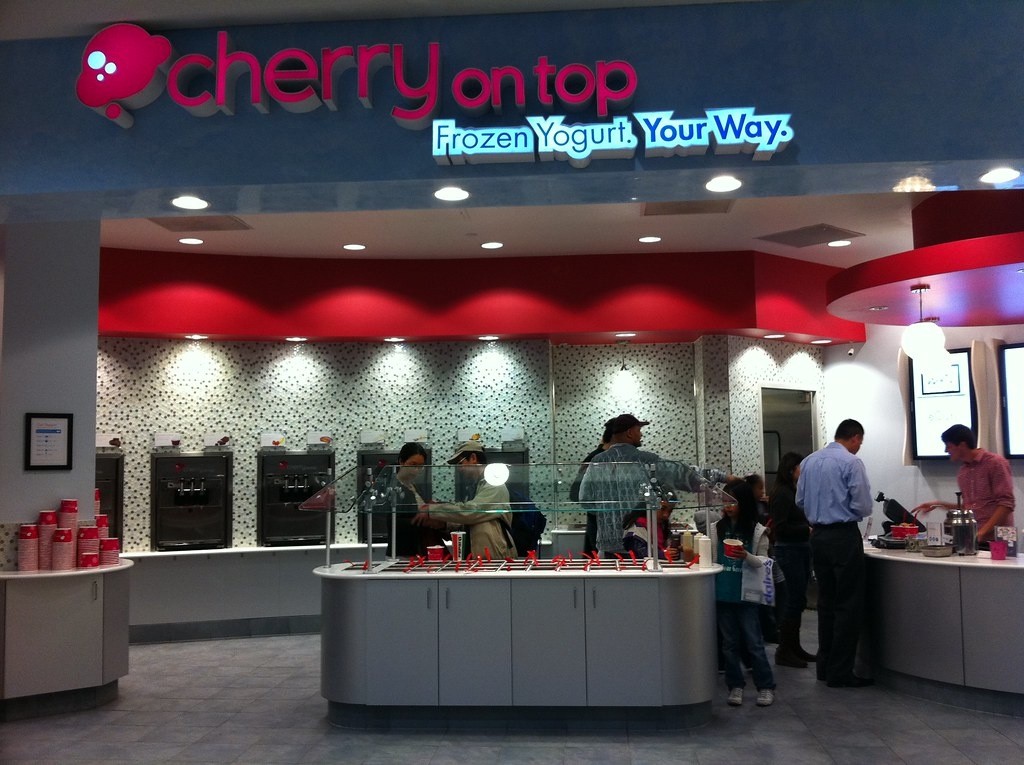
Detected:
[569, 414, 741, 561]
[768, 452, 816, 668]
[911, 425, 1015, 551]
[707, 474, 776, 707]
[795, 419, 875, 689]
[386, 442, 453, 560]
[411, 441, 518, 560]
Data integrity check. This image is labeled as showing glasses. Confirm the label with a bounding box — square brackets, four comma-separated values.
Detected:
[456, 457, 468, 468]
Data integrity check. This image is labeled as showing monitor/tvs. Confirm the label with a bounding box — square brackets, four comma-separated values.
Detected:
[883, 498, 926, 533]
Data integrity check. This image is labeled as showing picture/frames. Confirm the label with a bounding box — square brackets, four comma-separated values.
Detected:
[24, 412, 73, 471]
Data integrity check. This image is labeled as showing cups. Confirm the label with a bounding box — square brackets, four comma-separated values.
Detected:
[989, 540, 1008, 560]
[451, 532, 466, 561]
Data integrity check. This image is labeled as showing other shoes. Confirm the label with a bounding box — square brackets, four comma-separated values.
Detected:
[817, 663, 876, 687]
[718, 647, 727, 673]
[739, 646, 753, 669]
[760, 614, 785, 643]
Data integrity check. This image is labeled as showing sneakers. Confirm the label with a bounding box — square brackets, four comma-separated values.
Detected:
[756, 688, 775, 706]
[727, 687, 743, 705]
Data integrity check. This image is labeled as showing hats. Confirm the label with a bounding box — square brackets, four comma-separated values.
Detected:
[447, 439, 486, 465]
[611, 415, 650, 435]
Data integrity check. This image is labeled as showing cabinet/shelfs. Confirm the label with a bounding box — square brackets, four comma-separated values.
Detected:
[5, 574, 103, 698]
[514, 579, 662, 707]
[367, 579, 511, 707]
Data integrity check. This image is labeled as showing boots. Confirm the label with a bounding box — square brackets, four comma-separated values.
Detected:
[793, 624, 817, 662]
[775, 623, 808, 668]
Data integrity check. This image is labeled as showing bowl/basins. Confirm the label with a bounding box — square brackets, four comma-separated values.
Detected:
[891, 524, 918, 539]
[723, 539, 743, 558]
[920, 545, 952, 558]
[427, 546, 444, 560]
[18, 488, 120, 569]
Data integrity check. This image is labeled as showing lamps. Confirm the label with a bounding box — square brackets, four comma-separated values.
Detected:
[902, 283, 947, 356]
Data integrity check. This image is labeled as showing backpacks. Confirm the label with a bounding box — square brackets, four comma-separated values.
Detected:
[497, 485, 547, 558]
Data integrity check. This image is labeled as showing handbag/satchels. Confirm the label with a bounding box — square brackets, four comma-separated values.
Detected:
[740, 554, 776, 607]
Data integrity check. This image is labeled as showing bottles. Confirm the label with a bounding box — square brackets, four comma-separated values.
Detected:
[699, 535, 712, 568]
[683, 529, 693, 561]
[671, 529, 681, 561]
[963, 510, 977, 555]
[951, 509, 964, 556]
[943, 511, 951, 536]
[694, 532, 704, 563]
